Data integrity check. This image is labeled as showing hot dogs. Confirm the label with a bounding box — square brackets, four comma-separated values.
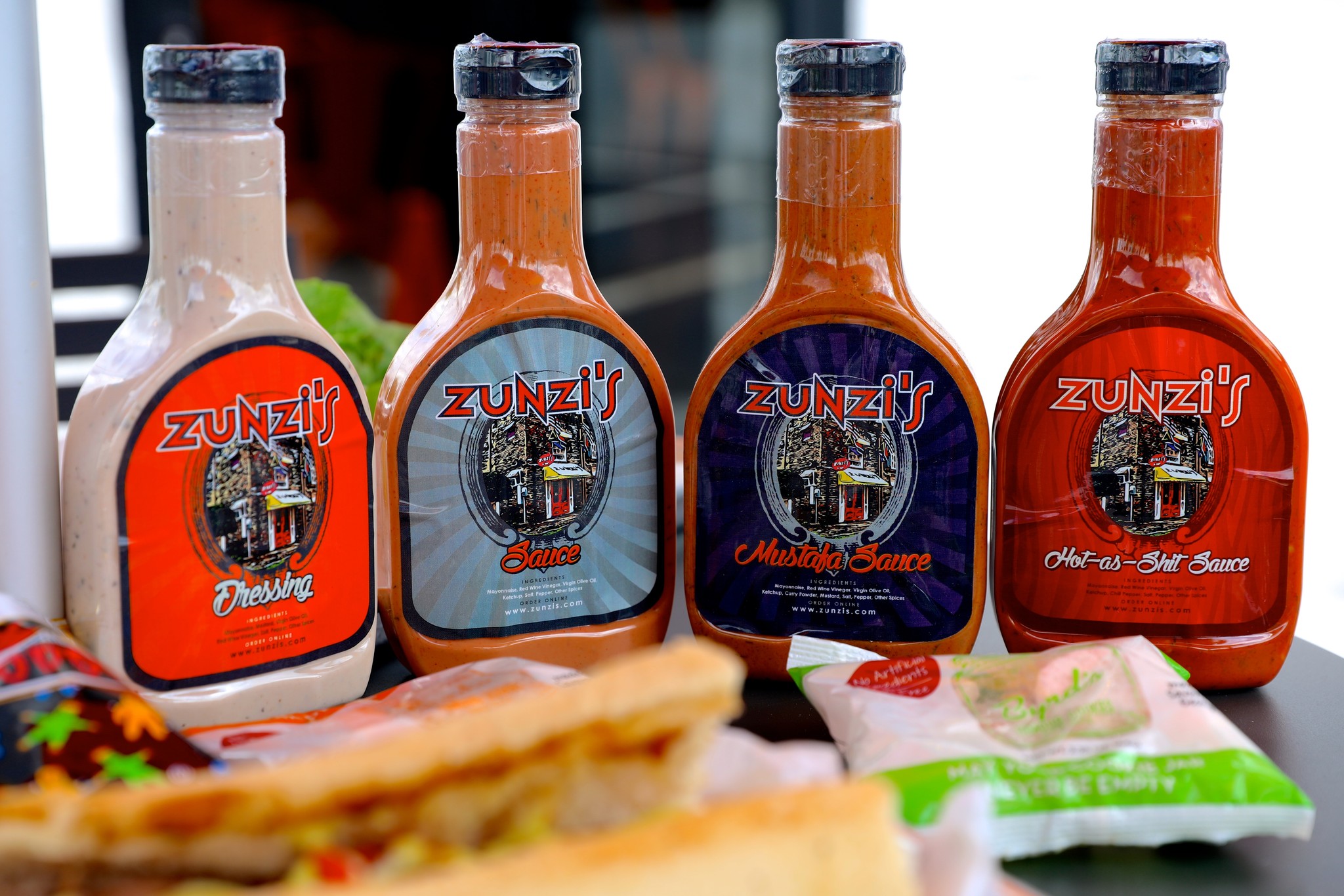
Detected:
[0, 632, 929, 896]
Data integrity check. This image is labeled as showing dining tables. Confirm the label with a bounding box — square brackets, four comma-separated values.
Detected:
[374, 497, 1344, 896]
